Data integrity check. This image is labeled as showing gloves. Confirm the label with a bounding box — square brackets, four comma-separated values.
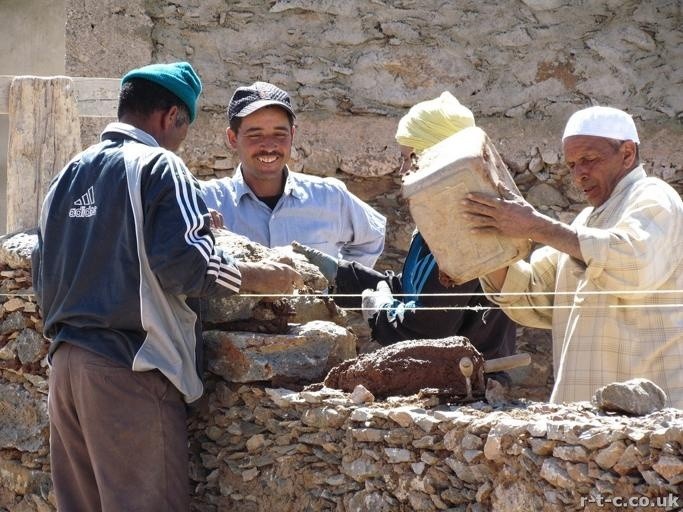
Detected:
[361, 280, 394, 326]
[291, 240, 339, 287]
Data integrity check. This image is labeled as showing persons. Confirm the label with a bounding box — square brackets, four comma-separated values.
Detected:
[32, 62, 305, 511]
[292, 92, 517, 389]
[459, 107, 683, 408]
[198, 81, 387, 295]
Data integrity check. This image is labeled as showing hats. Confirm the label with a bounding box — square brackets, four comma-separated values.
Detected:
[227, 81, 296, 125]
[121, 62, 202, 124]
[562, 106, 641, 146]
